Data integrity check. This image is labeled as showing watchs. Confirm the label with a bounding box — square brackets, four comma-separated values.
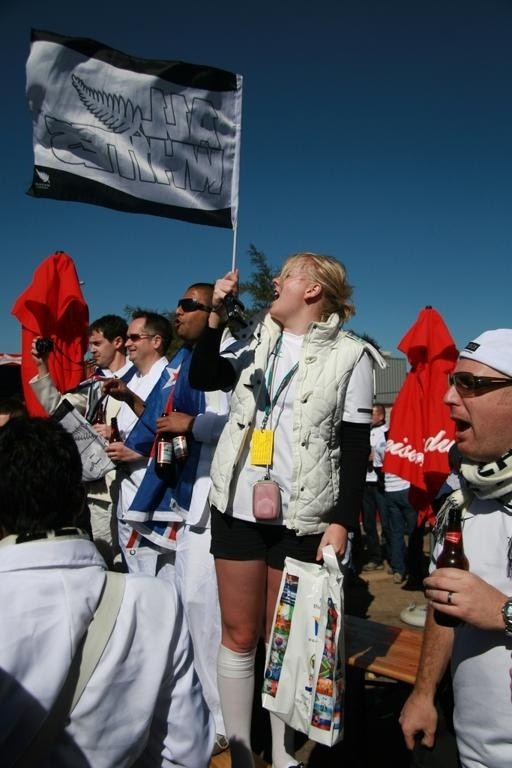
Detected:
[501, 598, 512, 632]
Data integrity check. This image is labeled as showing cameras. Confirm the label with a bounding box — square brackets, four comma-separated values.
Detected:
[36, 335, 55, 360]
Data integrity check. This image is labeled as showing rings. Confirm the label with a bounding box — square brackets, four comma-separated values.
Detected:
[448, 592, 452, 603]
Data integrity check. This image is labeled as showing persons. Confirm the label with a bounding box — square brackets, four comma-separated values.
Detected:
[1, 252, 386, 768]
[361, 403, 424, 584]
[399, 328, 512, 768]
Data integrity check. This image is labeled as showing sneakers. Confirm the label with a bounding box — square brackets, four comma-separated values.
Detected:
[393, 572, 403, 583]
[362, 562, 384, 571]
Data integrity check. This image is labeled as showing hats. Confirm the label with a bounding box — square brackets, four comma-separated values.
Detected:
[457, 329, 512, 377]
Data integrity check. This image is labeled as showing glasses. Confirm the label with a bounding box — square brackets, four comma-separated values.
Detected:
[447, 371, 510, 397]
[178, 298, 211, 312]
[126, 334, 153, 341]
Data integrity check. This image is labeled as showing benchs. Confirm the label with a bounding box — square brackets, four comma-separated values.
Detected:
[334, 615, 423, 714]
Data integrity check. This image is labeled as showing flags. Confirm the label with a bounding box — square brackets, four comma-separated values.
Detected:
[22, 29, 241, 234]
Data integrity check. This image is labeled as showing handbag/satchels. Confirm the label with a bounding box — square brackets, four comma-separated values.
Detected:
[261, 545, 346, 747]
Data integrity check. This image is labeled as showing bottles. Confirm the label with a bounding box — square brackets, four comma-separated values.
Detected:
[172, 408, 192, 463]
[92, 403, 105, 426]
[431, 507, 468, 628]
[154, 413, 174, 478]
[109, 416, 125, 463]
